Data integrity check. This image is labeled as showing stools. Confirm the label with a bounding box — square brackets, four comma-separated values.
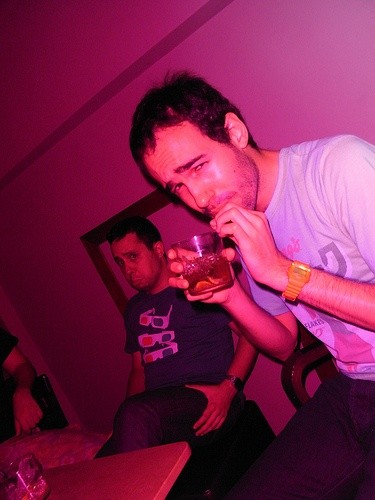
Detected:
[165, 398, 276, 500]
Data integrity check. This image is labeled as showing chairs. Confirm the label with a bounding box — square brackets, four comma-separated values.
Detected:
[279, 339, 335, 410]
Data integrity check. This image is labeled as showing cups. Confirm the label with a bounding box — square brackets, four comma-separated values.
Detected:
[0, 452, 51, 500]
[175, 234, 234, 296]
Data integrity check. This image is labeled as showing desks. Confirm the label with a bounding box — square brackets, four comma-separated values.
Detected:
[0, 441, 192, 500]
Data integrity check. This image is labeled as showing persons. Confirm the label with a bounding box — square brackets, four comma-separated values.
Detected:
[93, 215, 260, 459]
[129, 70, 375, 499]
[0, 320, 43, 444]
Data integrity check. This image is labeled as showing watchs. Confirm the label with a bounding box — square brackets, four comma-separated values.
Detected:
[222, 374, 244, 393]
[282, 260, 312, 303]
